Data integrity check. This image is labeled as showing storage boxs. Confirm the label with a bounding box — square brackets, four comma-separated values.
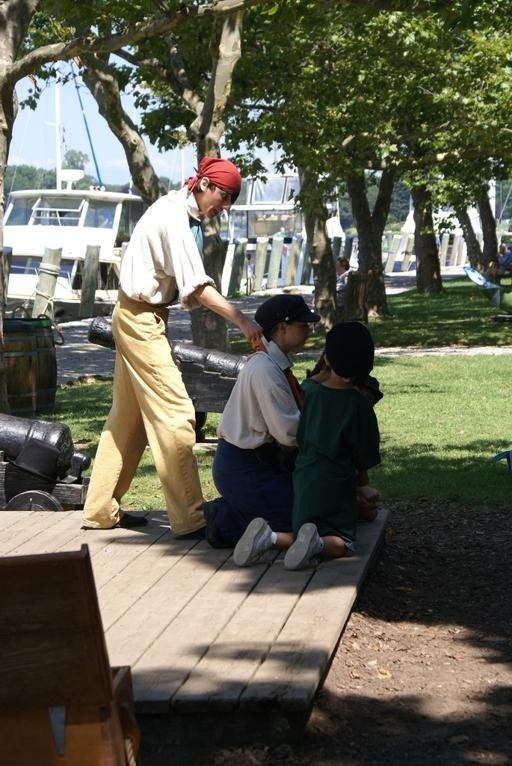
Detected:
[0, 544, 142, 765]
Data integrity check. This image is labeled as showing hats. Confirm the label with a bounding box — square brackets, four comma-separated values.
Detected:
[327, 321, 374, 378]
[255, 294, 321, 330]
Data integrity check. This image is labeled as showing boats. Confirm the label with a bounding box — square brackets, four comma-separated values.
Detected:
[217, 170, 360, 283]
[2, 168, 149, 323]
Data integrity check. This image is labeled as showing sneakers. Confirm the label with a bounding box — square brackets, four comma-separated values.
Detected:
[232, 517, 272, 566]
[111, 513, 149, 528]
[283, 522, 320, 570]
[202, 501, 227, 549]
[176, 525, 207, 540]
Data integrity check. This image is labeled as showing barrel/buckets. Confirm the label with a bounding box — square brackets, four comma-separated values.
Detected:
[1, 317, 57, 416]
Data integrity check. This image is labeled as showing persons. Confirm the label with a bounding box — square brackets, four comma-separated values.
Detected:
[203, 295, 320, 546]
[334, 257, 352, 311]
[233, 321, 383, 572]
[79, 158, 265, 540]
[497, 239, 511, 275]
